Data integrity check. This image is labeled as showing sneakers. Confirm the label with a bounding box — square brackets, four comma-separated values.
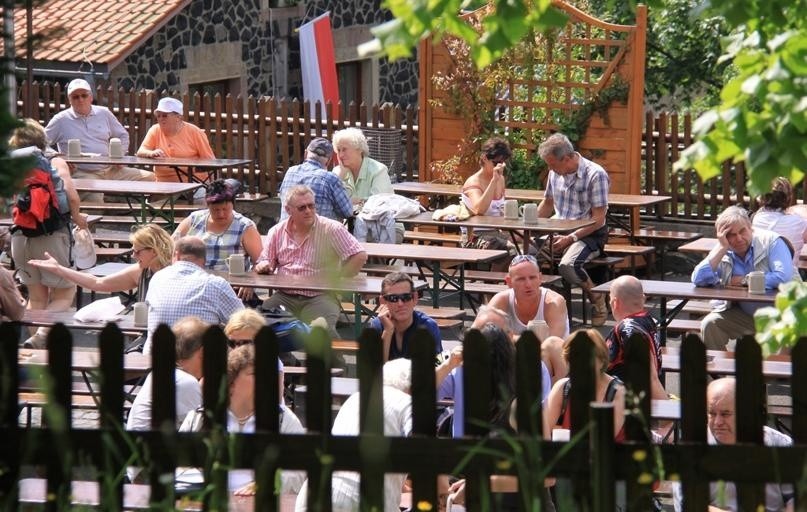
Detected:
[591, 292, 608, 327]
[23, 334, 47, 349]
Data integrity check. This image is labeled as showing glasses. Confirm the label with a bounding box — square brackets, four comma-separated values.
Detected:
[295, 203, 315, 212]
[227, 339, 254, 348]
[132, 246, 154, 255]
[382, 293, 412, 303]
[509, 255, 538, 265]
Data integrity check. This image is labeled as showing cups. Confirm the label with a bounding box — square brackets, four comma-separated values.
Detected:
[225, 253, 245, 277]
[106, 138, 122, 157]
[499, 199, 519, 221]
[133, 301, 147, 327]
[745, 271, 766, 295]
[67, 138, 80, 156]
[519, 204, 538, 223]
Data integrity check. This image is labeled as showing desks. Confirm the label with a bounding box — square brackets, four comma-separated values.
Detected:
[0, 155, 793, 420]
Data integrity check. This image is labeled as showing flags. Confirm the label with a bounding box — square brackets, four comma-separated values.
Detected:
[294, 11, 339, 134]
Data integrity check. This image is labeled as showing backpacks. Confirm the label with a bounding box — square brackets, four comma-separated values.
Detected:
[6, 146, 70, 237]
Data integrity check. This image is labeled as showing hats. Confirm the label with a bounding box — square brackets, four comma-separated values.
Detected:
[307, 137, 334, 157]
[71, 224, 97, 270]
[67, 78, 91, 95]
[153, 96, 184, 116]
